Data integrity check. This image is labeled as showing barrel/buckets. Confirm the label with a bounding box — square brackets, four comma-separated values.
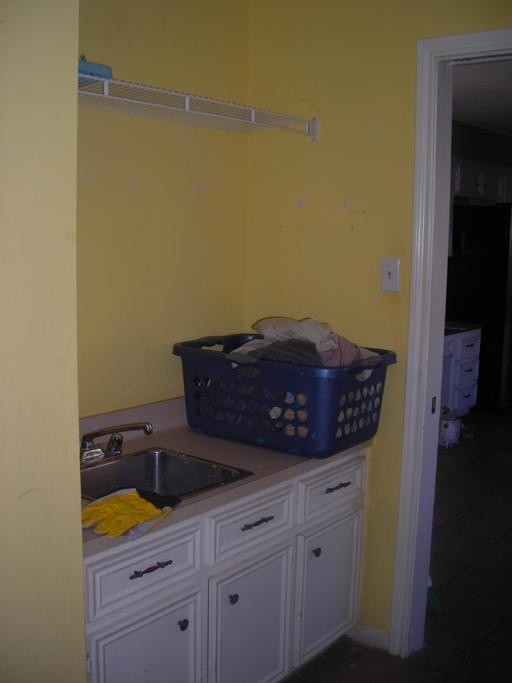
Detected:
[440, 414, 461, 447]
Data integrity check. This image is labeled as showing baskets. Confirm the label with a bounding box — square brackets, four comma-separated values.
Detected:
[172, 333, 396, 459]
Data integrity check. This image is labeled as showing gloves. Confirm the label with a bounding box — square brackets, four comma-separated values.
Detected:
[82, 487, 181, 541]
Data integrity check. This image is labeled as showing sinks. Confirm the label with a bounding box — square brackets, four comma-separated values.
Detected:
[81, 446, 260, 516]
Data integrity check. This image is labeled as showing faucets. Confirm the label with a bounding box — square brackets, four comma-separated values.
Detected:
[82, 422, 153, 439]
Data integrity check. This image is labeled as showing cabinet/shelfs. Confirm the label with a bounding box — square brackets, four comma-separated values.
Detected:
[83, 470, 297, 683]
[297, 441, 366, 668]
[440, 320, 481, 415]
